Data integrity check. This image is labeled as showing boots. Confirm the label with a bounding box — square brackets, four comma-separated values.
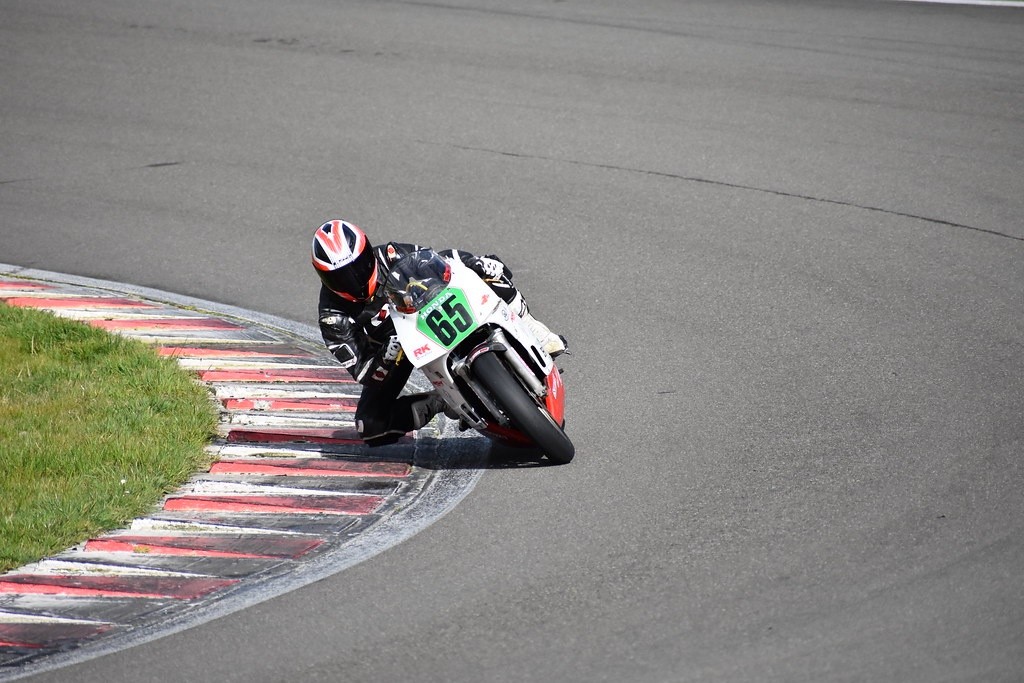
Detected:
[401, 388, 460, 430]
[506, 287, 567, 357]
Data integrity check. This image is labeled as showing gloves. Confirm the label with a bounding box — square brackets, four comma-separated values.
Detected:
[465, 256, 505, 282]
[375, 335, 403, 372]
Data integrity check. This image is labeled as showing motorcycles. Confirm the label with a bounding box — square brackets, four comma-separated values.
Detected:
[382, 251, 575, 465]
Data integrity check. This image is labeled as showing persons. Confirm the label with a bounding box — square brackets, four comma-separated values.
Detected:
[311, 218, 568, 447]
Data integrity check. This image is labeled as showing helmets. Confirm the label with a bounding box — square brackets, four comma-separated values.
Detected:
[311, 218, 377, 304]
